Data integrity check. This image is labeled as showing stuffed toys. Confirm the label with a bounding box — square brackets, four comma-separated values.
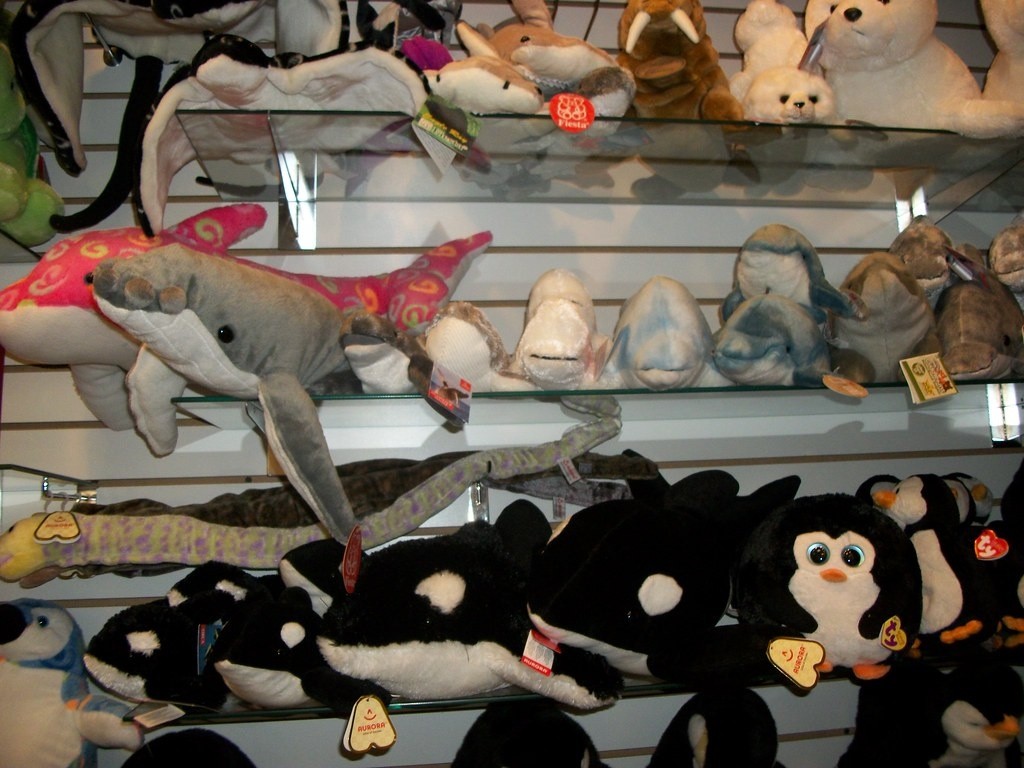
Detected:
[1, 1, 1024, 767]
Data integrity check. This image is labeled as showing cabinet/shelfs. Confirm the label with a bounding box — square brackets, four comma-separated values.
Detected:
[0, 223, 85, 494]
[168, 107, 1024, 727]
